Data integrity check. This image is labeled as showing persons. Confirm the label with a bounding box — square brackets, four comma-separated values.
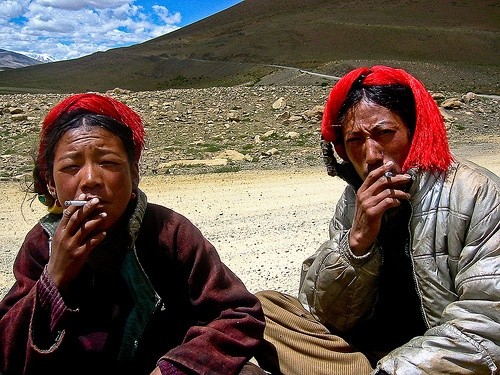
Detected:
[0, 93, 267, 375]
[253, 66, 500, 375]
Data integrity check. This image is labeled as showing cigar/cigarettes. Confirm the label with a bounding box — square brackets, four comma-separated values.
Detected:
[384, 172, 393, 178]
[65, 201, 89, 207]
